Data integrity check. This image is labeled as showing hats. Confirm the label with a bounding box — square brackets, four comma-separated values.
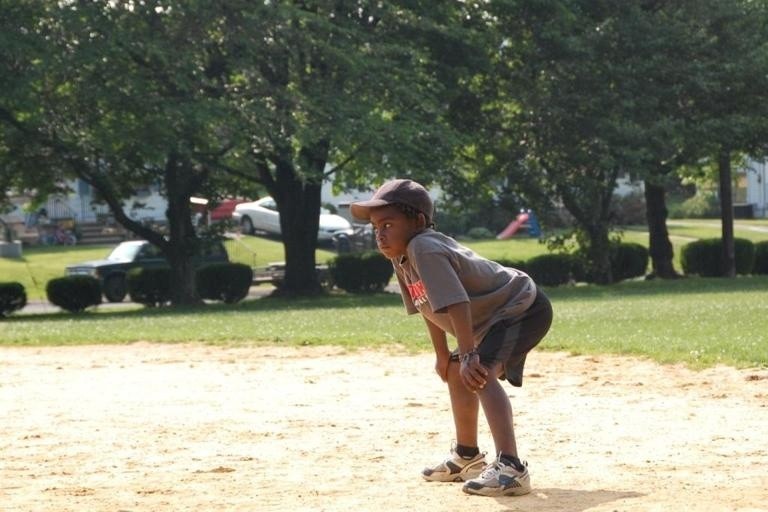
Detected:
[350, 179, 434, 223]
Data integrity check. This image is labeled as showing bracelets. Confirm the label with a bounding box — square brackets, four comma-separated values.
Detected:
[458, 348, 478, 364]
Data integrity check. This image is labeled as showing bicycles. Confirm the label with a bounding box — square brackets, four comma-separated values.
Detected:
[39, 221, 78, 246]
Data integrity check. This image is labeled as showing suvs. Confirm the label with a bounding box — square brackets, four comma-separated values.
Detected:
[65, 235, 230, 303]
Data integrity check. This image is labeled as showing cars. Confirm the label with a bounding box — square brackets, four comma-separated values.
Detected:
[190, 192, 252, 226]
[233, 196, 353, 246]
[123, 190, 206, 223]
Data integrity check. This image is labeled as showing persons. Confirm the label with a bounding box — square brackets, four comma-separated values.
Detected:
[350, 178, 552, 498]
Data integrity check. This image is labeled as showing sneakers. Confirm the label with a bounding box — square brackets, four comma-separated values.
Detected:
[461, 460, 533, 496]
[419, 448, 488, 482]
